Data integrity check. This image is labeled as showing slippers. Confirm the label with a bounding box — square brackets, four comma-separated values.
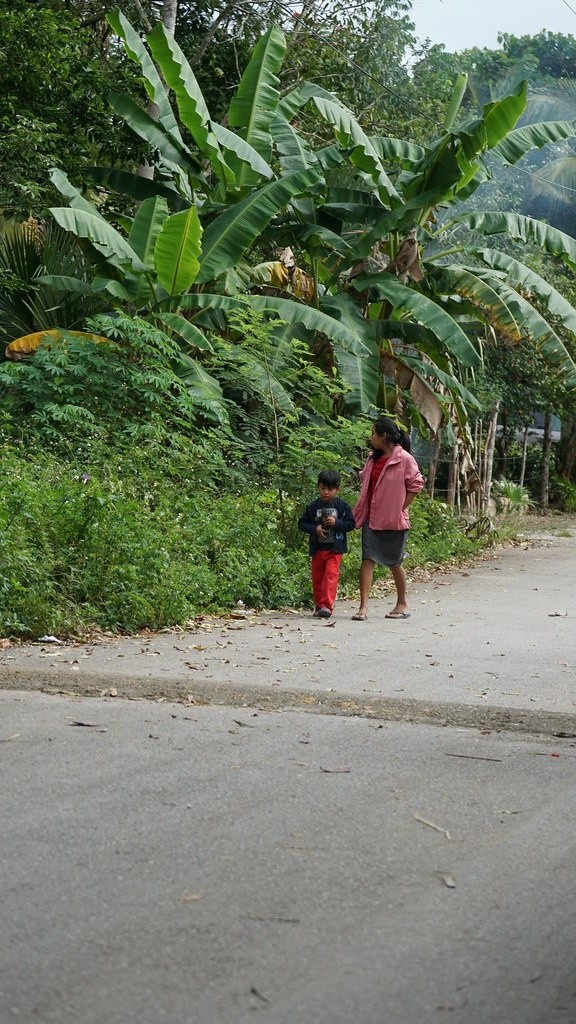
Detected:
[353, 611, 367, 620]
[383, 610, 410, 620]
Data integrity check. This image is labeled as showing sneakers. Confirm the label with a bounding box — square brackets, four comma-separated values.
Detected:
[312, 607, 331, 618]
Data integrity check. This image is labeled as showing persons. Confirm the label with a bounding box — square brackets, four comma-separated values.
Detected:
[297, 470, 355, 617]
[352, 418, 424, 620]
